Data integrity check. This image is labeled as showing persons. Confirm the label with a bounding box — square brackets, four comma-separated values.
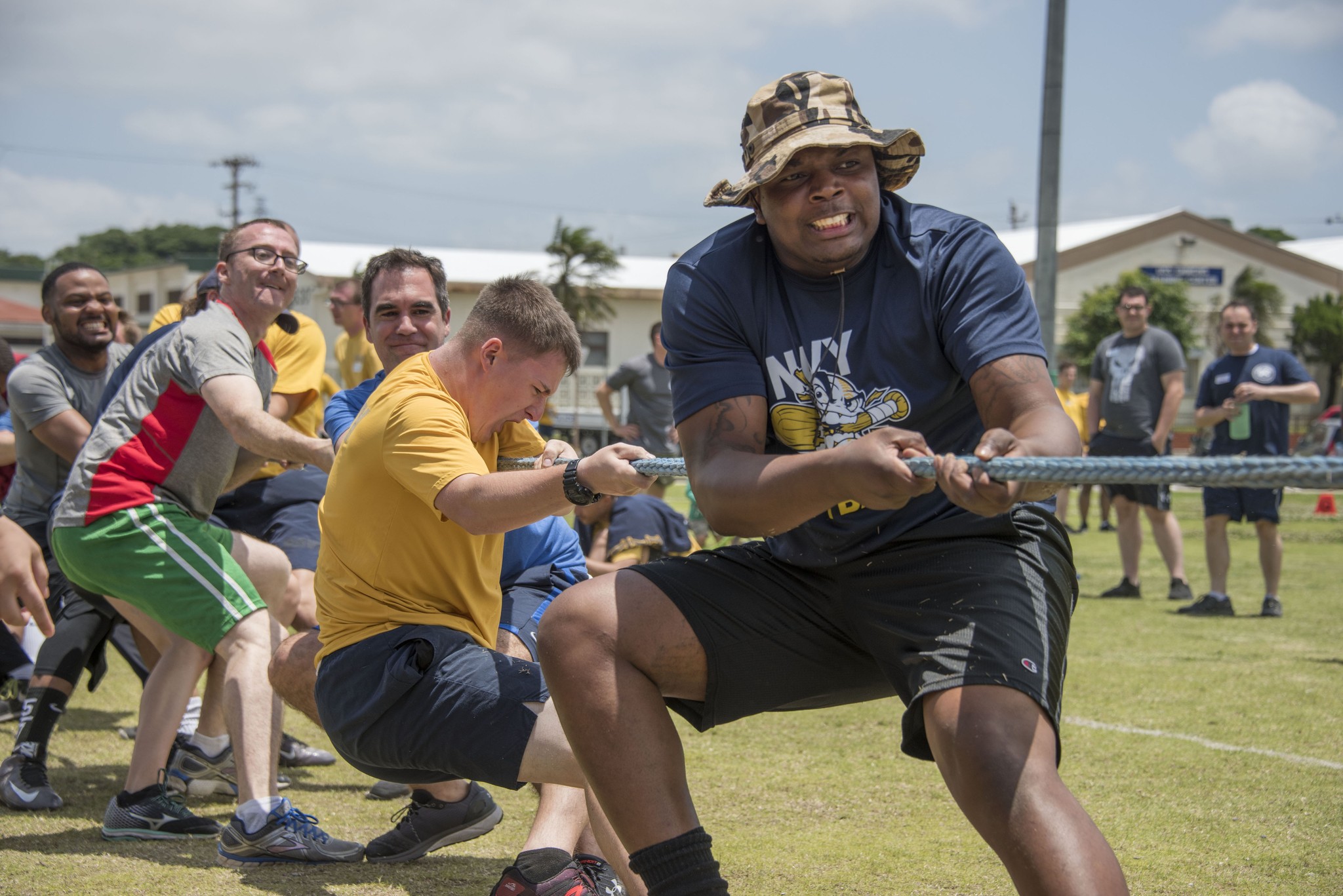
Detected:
[312, 274, 661, 896]
[535, 69, 1130, 896]
[0, 505, 56, 641]
[266, 245, 625, 895]
[1175, 297, 1322, 617]
[595, 321, 682, 499]
[46, 265, 222, 843]
[1054, 360, 1117, 534]
[686, 478, 726, 550]
[573, 491, 702, 578]
[0, 219, 383, 813]
[51, 219, 369, 864]
[1082, 284, 1195, 601]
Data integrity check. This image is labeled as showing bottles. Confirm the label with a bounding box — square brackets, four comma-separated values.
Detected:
[1229, 402, 1251, 440]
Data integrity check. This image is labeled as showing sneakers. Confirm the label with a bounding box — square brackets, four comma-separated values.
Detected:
[1103, 577, 1142, 599]
[1168, 578, 1193, 599]
[119, 728, 194, 748]
[368, 775, 411, 800]
[215, 796, 366, 866]
[276, 732, 337, 767]
[1, 675, 31, 723]
[489, 861, 594, 896]
[1179, 591, 1236, 616]
[574, 853, 626, 896]
[367, 777, 505, 861]
[101, 785, 222, 839]
[1261, 598, 1281, 618]
[165, 744, 293, 800]
[0, 753, 64, 810]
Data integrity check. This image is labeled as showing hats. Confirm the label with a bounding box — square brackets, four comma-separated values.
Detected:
[706, 71, 922, 209]
[196, 270, 298, 336]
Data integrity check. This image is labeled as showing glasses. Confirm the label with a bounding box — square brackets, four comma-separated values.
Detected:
[225, 248, 308, 275]
[328, 298, 363, 310]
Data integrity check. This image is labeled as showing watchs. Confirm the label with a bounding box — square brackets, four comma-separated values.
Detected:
[561, 457, 602, 507]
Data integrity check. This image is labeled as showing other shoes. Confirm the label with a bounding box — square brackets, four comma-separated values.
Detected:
[1078, 521, 1087, 532]
[1099, 522, 1117, 531]
